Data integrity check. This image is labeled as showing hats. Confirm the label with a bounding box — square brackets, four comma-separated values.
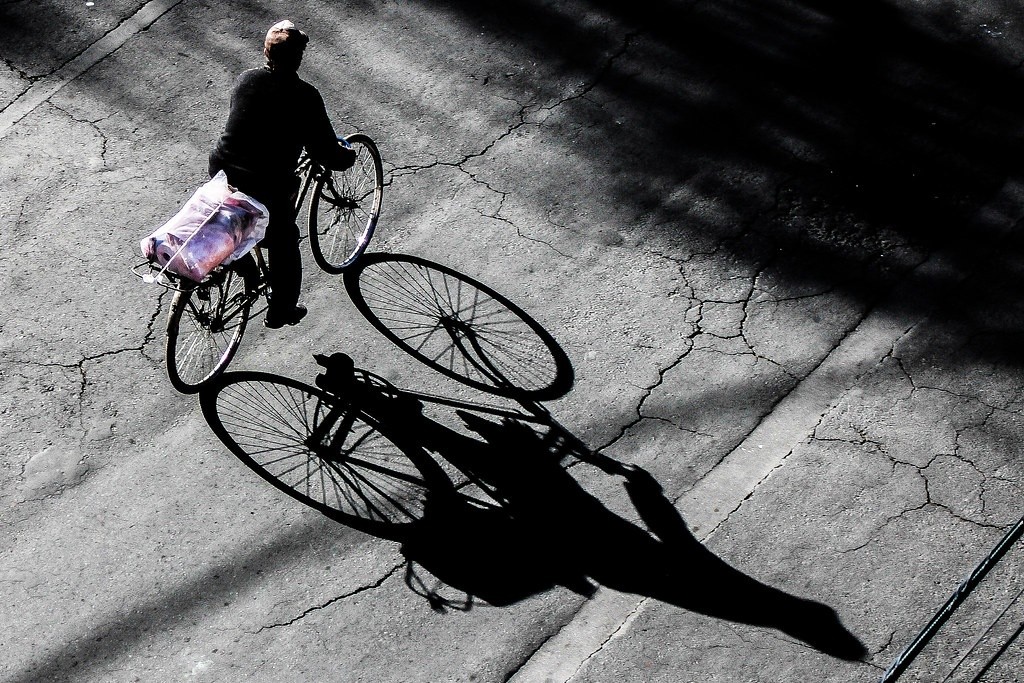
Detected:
[264, 20, 309, 61]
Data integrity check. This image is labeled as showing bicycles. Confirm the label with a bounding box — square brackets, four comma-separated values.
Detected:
[135, 133, 384, 395]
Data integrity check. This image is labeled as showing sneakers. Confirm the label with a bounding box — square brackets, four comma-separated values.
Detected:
[265, 304, 307, 329]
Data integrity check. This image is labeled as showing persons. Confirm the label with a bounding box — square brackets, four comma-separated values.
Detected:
[207, 19, 357, 329]
